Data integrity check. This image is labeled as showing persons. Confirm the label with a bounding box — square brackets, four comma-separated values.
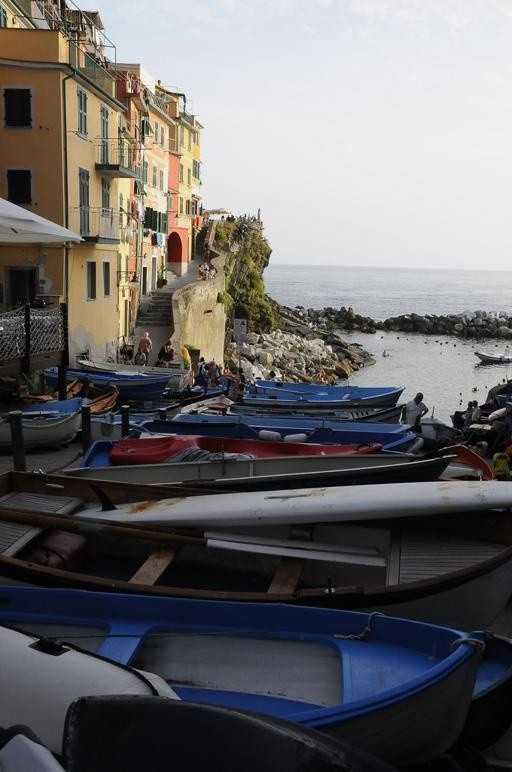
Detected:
[202, 216, 212, 227]
[138, 332, 152, 365]
[135, 347, 146, 367]
[158, 341, 173, 362]
[220, 214, 235, 222]
[196, 263, 217, 281]
[196, 357, 245, 398]
[270, 371, 276, 381]
[463, 401, 472, 426]
[472, 400, 480, 425]
[401, 393, 428, 434]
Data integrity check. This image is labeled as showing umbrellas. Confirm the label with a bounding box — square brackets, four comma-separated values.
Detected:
[204, 208, 227, 223]
[0, 196, 85, 246]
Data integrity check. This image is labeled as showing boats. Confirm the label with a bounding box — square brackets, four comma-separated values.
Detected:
[475, 352, 512, 364]
